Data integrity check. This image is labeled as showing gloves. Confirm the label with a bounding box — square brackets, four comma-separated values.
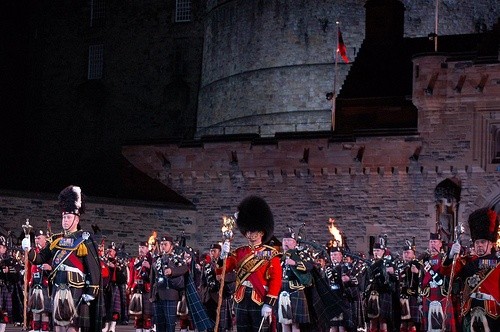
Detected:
[448, 244, 461, 257]
[220, 242, 231, 257]
[82, 294, 94, 301]
[260, 303, 272, 320]
[21, 238, 31, 253]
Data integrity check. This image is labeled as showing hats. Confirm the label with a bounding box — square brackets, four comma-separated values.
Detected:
[140, 240, 149, 247]
[0, 236, 7, 246]
[236, 195, 274, 245]
[210, 244, 221, 250]
[429, 233, 441, 240]
[373, 243, 386, 250]
[161, 237, 172, 242]
[330, 247, 342, 253]
[468, 207, 499, 242]
[35, 229, 46, 235]
[107, 245, 118, 252]
[403, 245, 413, 251]
[283, 233, 296, 240]
[58, 186, 87, 217]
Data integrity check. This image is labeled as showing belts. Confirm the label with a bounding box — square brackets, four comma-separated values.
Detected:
[58, 264, 87, 280]
[471, 293, 495, 301]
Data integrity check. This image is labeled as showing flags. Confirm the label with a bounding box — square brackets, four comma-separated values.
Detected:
[337, 26, 349, 65]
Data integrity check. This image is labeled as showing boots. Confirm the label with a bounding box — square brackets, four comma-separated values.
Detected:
[108, 321, 117, 332]
[0, 322, 6, 332]
[102, 322, 109, 332]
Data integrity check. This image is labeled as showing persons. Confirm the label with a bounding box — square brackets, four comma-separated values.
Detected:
[0, 230, 500, 332]
[22, 186, 106, 332]
[216, 195, 283, 332]
[439, 208, 500, 332]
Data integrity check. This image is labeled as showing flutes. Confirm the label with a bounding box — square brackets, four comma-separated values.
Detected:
[283, 223, 329, 279]
[334, 239, 382, 298]
[374, 235, 445, 302]
